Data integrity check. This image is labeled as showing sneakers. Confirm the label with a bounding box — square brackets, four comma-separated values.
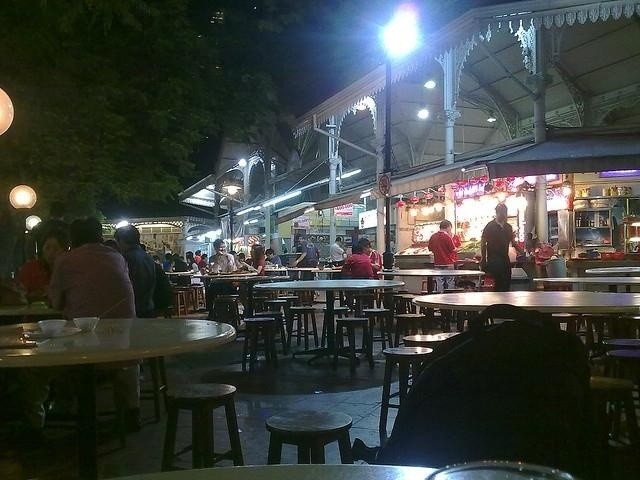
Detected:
[123, 411, 142, 431]
[28, 405, 46, 437]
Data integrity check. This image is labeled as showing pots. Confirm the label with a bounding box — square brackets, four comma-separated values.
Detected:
[625, 241, 639, 253]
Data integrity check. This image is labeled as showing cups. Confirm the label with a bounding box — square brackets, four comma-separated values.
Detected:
[39, 320, 67, 331]
[574, 187, 591, 198]
[603, 187, 632, 198]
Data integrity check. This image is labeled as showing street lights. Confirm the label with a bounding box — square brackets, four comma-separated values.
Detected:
[10, 183, 40, 301]
[0, 88, 16, 137]
[376, 8, 425, 312]
[223, 182, 241, 252]
[24, 214, 44, 260]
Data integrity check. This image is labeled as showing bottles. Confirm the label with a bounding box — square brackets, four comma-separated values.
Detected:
[576, 212, 604, 227]
[585, 248, 601, 259]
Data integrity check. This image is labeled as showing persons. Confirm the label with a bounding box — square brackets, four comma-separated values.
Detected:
[20, 228, 69, 299]
[21, 218, 133, 439]
[428, 218, 457, 293]
[480, 203, 528, 290]
[104, 208, 386, 323]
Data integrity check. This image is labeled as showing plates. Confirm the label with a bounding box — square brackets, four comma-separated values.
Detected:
[26, 327, 82, 338]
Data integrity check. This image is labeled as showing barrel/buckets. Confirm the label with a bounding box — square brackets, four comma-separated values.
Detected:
[544, 257, 567, 278]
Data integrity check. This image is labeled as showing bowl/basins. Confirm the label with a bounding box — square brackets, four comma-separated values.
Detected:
[71, 332, 100, 346]
[572, 199, 612, 210]
[36, 343, 67, 354]
[73, 318, 99, 332]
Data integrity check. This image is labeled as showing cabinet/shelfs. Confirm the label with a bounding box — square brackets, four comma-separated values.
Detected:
[548, 197, 639, 253]
